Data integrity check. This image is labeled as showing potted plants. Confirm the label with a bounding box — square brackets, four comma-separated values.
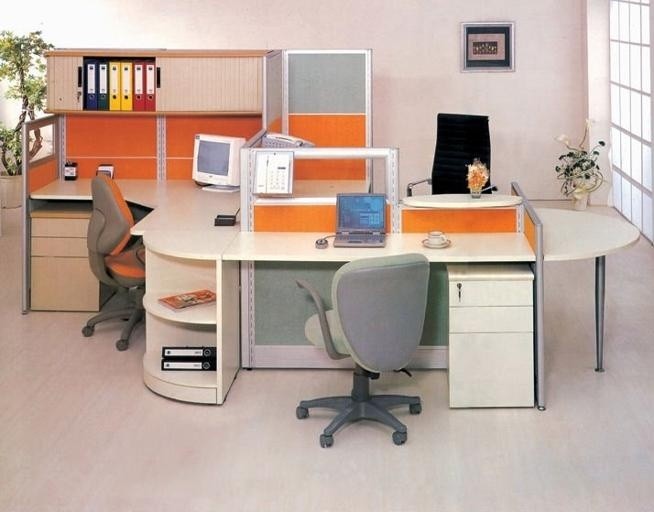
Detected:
[0, 27, 57, 211]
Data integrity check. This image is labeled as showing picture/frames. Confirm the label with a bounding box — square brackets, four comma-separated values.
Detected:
[459, 21, 516, 74]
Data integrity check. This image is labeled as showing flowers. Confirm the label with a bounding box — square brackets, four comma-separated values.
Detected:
[553, 117, 611, 207]
[464, 157, 490, 193]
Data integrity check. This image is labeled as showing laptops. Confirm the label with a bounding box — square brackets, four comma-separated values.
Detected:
[333, 194, 387, 248]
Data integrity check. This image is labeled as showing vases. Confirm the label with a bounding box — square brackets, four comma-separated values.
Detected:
[571, 190, 588, 210]
[469, 186, 482, 199]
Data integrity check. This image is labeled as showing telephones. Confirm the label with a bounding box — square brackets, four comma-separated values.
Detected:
[254, 151, 294, 196]
[260, 133, 316, 150]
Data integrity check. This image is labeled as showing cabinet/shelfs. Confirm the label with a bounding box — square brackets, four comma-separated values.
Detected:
[445, 264, 536, 410]
[42, 48, 274, 117]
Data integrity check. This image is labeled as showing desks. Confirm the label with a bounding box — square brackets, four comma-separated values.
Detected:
[530, 207, 642, 372]
[251, 179, 371, 200]
[28, 201, 116, 314]
[401, 193, 524, 208]
[221, 231, 538, 265]
[28, 176, 241, 410]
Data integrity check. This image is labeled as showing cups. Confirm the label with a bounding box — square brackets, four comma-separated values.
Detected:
[427, 232, 447, 246]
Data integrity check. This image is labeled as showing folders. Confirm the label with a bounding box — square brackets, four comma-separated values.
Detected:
[161, 347, 217, 371]
[86, 60, 156, 111]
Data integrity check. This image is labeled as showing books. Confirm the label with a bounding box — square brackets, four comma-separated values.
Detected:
[158, 289, 217, 313]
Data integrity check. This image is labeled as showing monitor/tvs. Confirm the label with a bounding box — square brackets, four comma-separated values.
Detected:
[191, 134, 246, 192]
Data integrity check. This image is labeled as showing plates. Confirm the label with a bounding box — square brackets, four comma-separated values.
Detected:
[421, 238, 450, 249]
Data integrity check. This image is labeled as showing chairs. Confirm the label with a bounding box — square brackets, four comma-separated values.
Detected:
[80, 172, 145, 352]
[405, 112, 498, 198]
[293, 251, 432, 448]
